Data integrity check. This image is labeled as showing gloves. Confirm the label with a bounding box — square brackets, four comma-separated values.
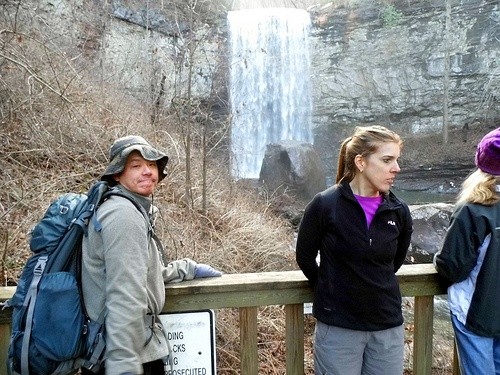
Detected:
[193, 262, 222, 278]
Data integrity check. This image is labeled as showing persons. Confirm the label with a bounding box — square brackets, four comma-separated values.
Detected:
[434, 127, 500, 375]
[77, 134, 222, 375]
[295, 125, 414, 375]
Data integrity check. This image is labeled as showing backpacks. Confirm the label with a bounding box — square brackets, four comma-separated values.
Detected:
[0, 181, 160, 375]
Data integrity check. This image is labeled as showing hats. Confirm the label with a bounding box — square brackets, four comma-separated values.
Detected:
[100, 135, 169, 186]
[475, 128, 500, 176]
